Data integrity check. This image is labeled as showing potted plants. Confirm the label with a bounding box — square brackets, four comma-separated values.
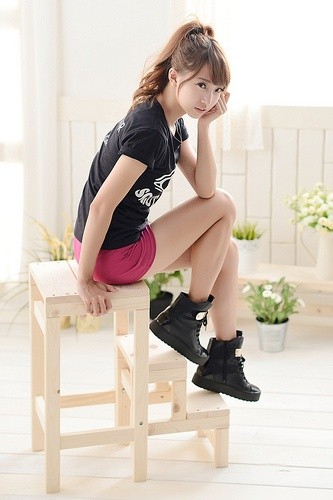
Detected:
[145, 270, 185, 320]
[232, 219, 263, 263]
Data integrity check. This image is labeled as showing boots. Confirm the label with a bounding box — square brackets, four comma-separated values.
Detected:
[149, 292, 215, 366]
[191, 330, 261, 402]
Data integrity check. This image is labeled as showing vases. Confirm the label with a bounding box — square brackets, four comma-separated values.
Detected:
[254, 316, 289, 353]
[313, 229, 333, 282]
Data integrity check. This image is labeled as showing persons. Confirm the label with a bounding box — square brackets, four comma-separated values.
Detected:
[73, 20, 261, 402]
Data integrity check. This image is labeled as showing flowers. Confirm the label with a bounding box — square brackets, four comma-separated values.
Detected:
[288, 181, 333, 233]
[241, 277, 304, 323]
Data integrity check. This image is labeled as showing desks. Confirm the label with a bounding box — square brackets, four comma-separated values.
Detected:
[235, 263, 333, 328]
